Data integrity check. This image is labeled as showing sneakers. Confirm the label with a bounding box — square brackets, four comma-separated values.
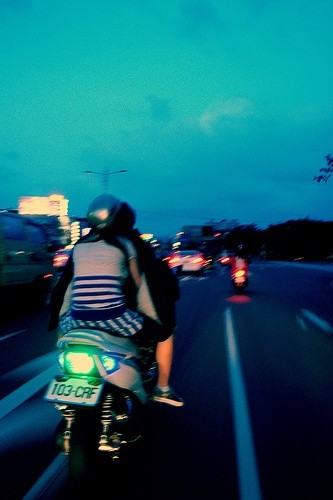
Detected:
[152, 387, 184, 407]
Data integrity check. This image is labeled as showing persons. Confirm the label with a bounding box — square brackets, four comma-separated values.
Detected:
[218, 248, 251, 283]
[43, 195, 188, 409]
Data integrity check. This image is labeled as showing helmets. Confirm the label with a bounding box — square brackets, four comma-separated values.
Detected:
[87, 192, 122, 230]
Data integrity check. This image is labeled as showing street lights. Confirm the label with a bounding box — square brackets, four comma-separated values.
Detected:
[84, 169, 128, 194]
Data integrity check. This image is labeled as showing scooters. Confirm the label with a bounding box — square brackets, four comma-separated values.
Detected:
[221, 255, 230, 266]
[231, 269, 249, 293]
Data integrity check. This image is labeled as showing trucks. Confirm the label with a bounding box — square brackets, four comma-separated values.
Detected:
[0, 209, 61, 335]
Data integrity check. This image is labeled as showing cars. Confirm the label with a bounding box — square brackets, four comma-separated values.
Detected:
[162, 250, 214, 276]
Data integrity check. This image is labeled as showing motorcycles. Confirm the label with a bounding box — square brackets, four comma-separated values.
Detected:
[44, 329, 151, 496]
